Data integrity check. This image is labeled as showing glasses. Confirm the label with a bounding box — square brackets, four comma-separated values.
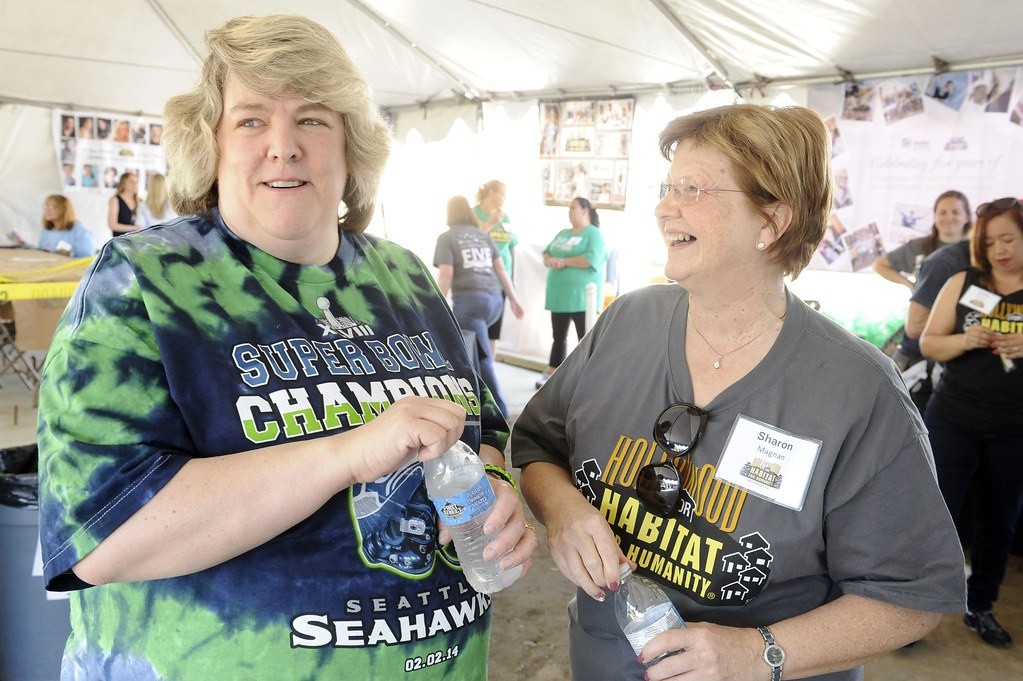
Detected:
[635, 402, 711, 519]
[657, 177, 751, 206]
[975, 196, 1018, 216]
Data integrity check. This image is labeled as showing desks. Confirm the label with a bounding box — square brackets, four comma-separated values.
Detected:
[0, 247, 94, 409]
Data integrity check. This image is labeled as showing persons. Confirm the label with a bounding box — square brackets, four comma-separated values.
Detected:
[540, 99, 632, 202]
[535, 198, 608, 389]
[8, 194, 94, 259]
[510, 106, 970, 681]
[60, 115, 163, 192]
[436, 179, 527, 365]
[109, 173, 178, 238]
[835, 68, 1023, 122]
[874, 191, 1023, 651]
[37, 19, 536, 681]
[821, 127, 925, 266]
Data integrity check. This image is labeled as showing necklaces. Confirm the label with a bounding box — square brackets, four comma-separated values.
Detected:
[687, 312, 787, 370]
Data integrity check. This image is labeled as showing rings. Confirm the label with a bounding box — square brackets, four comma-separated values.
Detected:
[526, 521, 536, 533]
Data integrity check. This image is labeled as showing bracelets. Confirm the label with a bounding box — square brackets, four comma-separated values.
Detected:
[484, 463, 520, 497]
[560, 259, 566, 268]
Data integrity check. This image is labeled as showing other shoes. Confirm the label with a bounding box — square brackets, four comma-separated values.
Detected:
[534, 371, 552, 389]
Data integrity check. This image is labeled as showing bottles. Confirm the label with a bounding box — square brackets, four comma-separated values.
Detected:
[614, 562, 688, 669]
[424, 439, 523, 594]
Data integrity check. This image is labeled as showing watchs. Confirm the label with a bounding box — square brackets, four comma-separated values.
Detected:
[757, 626, 785, 681]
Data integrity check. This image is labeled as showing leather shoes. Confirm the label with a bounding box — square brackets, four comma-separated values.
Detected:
[962, 610, 1014, 649]
